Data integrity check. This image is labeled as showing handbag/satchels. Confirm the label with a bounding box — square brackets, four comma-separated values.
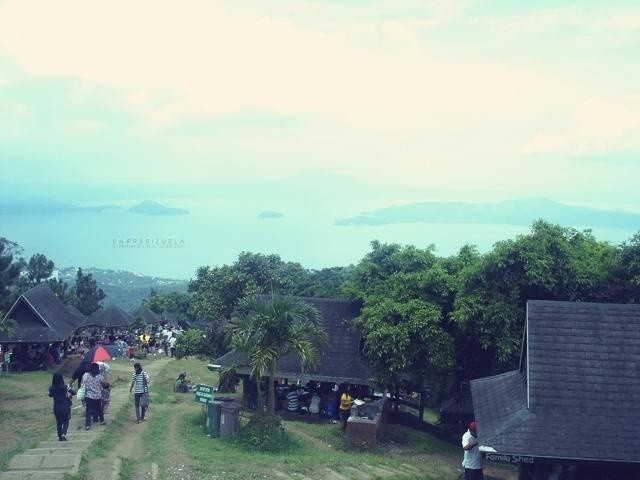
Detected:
[139, 393, 149, 407]
[77, 383, 85, 401]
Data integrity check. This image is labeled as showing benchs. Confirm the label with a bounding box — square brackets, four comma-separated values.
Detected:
[277, 410, 323, 423]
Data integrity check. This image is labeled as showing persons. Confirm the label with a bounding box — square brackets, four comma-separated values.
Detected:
[14, 324, 178, 369]
[69, 360, 110, 430]
[462, 422, 483, 480]
[283, 379, 356, 432]
[176, 371, 191, 393]
[129, 362, 150, 424]
[47, 372, 77, 441]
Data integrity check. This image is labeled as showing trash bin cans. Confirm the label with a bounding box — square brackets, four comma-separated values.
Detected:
[205, 401, 241, 438]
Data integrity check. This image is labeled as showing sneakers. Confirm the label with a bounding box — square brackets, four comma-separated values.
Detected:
[100, 421, 105, 425]
[59, 434, 66, 441]
[86, 426, 90, 429]
